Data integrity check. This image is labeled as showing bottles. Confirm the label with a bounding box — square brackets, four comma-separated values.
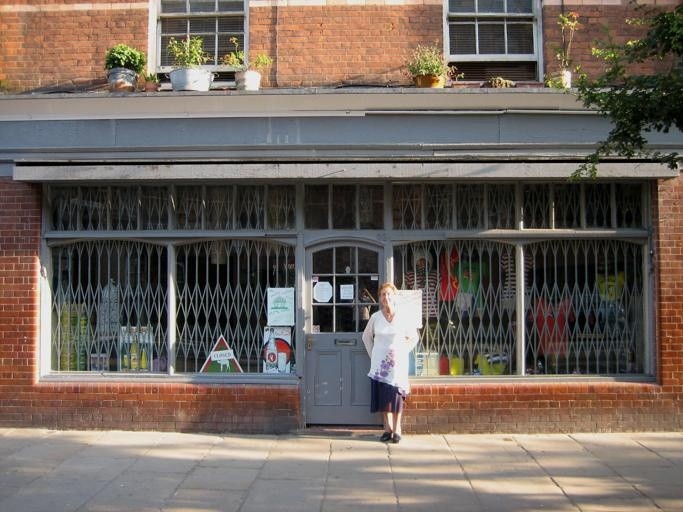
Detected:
[121, 338, 148, 369]
[266, 328, 277, 371]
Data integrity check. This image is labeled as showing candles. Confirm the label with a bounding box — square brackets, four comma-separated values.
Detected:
[404, 38, 465, 87]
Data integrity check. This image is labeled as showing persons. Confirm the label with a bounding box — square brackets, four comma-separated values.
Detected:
[403, 241, 488, 345]
[350, 285, 376, 333]
[528, 285, 575, 363]
[591, 257, 628, 324]
[363, 283, 419, 444]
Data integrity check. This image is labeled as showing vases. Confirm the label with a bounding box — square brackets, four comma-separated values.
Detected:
[546, 10, 585, 88]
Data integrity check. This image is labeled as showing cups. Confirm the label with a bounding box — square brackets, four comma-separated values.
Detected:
[277, 352, 287, 372]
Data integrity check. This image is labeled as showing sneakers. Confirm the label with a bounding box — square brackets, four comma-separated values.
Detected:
[378, 431, 403, 443]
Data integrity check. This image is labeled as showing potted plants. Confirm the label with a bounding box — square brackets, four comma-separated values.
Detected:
[482, 78, 517, 88]
[220, 37, 274, 90]
[168, 36, 214, 90]
[104, 44, 147, 93]
